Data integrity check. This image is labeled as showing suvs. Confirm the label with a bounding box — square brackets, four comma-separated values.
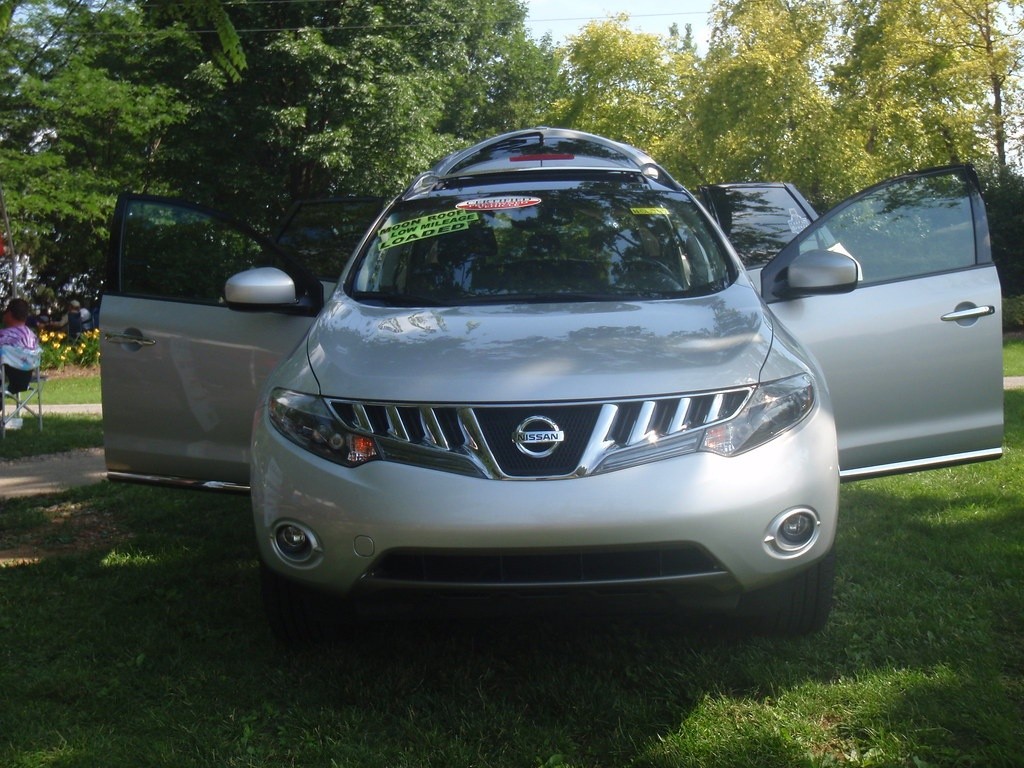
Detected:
[99, 125, 1004, 636]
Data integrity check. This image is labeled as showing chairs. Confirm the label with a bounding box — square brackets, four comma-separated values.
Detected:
[588, 211, 647, 271]
[0, 356, 48, 439]
[436, 226, 498, 296]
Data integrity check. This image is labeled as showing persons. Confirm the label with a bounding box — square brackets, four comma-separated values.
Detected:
[25, 311, 69, 329]
[0, 299, 38, 413]
[70, 301, 93, 329]
